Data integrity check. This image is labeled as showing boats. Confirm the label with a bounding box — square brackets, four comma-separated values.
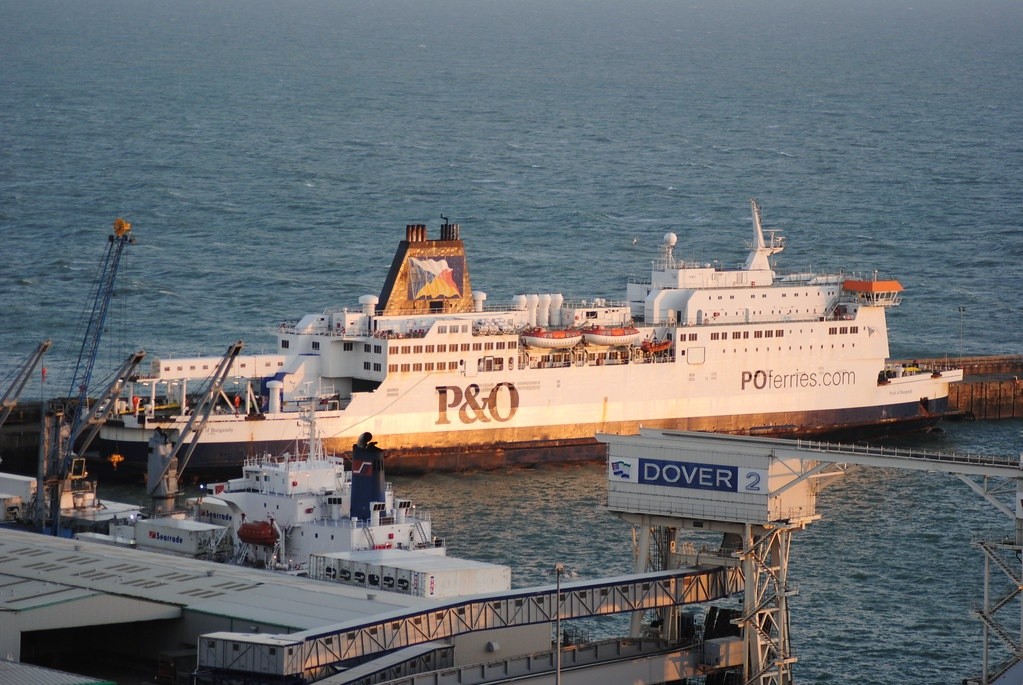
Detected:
[76, 200, 968, 482]
[0, 471, 149, 536]
[0, 337, 104, 464]
